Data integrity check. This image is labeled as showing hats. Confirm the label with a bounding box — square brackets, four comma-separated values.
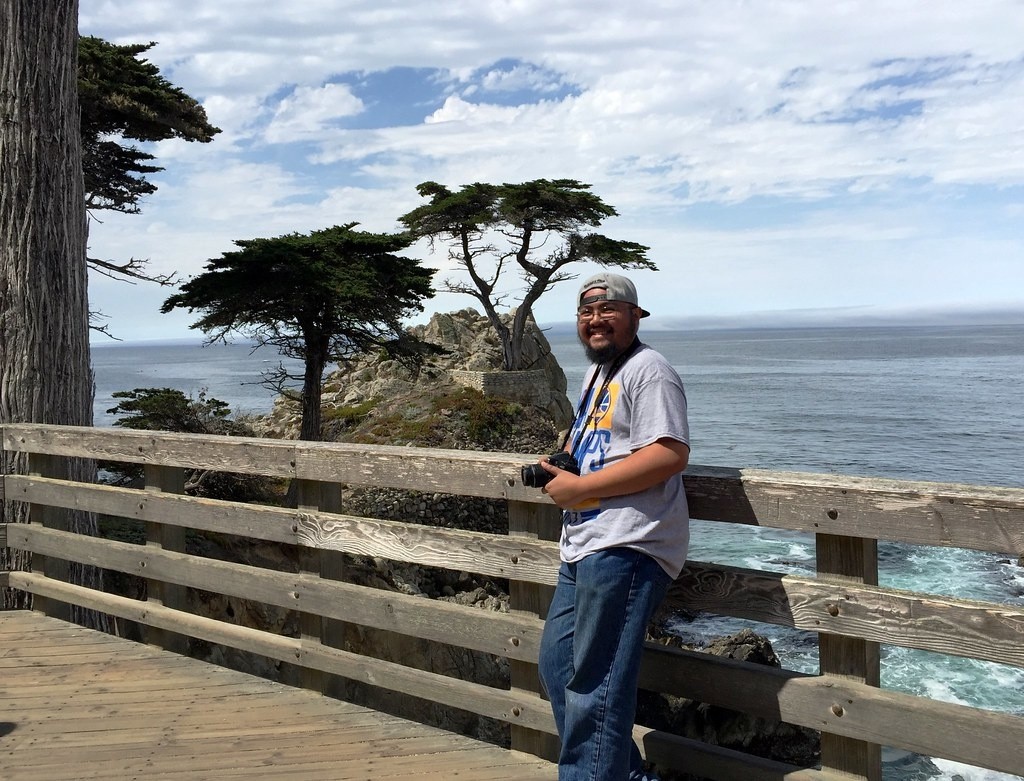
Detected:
[576, 273, 650, 318]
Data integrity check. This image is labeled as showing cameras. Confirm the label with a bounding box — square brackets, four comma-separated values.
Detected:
[522, 451, 581, 488]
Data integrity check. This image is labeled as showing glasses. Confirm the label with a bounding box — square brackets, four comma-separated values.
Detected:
[577, 305, 631, 321]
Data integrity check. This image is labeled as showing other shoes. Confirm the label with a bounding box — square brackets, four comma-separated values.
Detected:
[628, 766, 660, 781]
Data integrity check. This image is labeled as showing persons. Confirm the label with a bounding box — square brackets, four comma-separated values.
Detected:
[538, 274, 691, 781]
